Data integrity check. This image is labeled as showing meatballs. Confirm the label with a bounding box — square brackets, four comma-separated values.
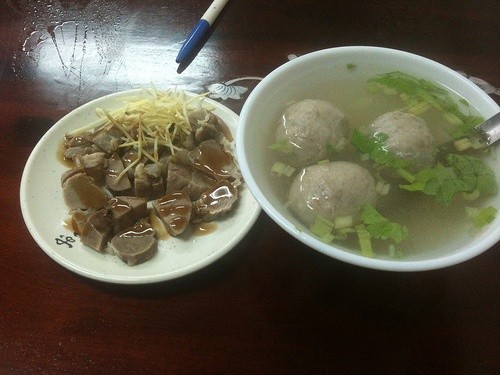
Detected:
[286, 159, 375, 228]
[275, 98, 355, 168]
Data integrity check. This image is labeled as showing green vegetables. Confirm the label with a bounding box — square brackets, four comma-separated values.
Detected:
[316, 69, 500, 251]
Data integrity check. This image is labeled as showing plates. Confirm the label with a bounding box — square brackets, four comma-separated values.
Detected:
[18, 87, 261, 285]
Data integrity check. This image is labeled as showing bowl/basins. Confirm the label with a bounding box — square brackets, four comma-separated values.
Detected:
[234, 46, 500, 272]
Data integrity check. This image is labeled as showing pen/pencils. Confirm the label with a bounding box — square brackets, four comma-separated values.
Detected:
[175, 0, 228, 65]
